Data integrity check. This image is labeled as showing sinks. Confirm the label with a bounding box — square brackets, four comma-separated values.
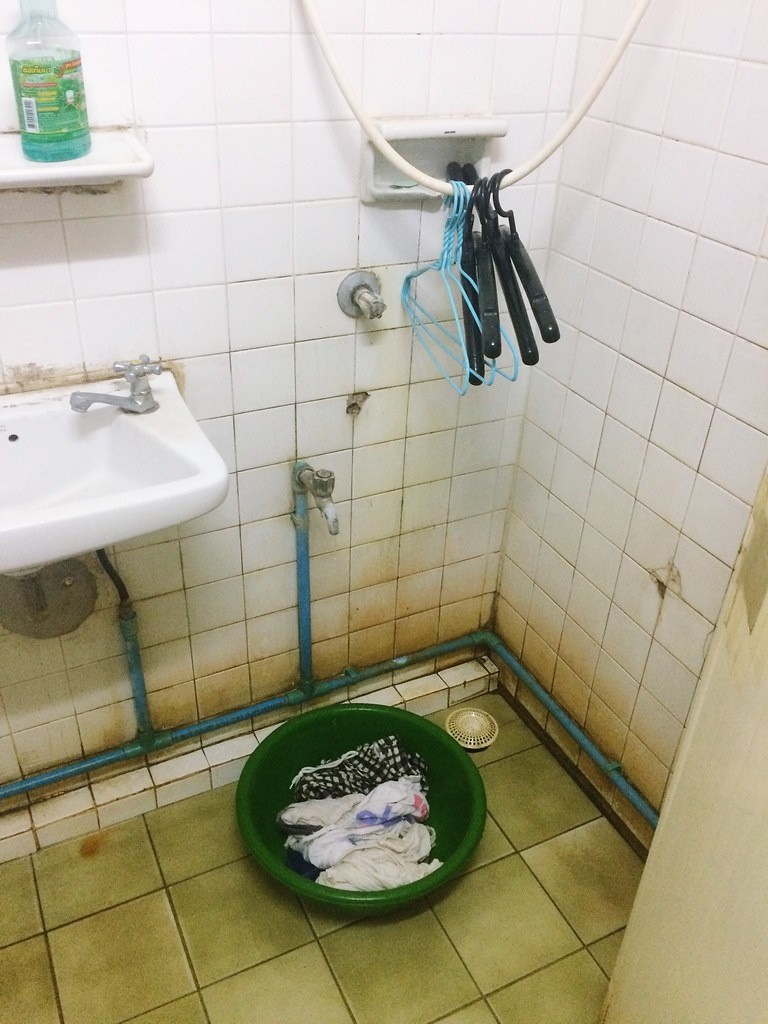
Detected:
[2, 368, 231, 581]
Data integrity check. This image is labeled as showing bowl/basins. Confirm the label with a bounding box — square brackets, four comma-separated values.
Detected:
[235, 702, 488, 914]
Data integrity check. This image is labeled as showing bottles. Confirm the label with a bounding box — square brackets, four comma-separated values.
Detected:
[7, 0, 91, 163]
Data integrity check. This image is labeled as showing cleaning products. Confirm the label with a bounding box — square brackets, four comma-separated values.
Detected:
[6, 0, 91, 166]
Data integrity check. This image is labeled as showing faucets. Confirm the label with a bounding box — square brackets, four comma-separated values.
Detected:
[71, 354, 162, 419]
[299, 464, 342, 535]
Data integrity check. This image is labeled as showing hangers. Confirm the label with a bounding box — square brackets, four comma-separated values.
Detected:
[402, 167, 561, 395]
